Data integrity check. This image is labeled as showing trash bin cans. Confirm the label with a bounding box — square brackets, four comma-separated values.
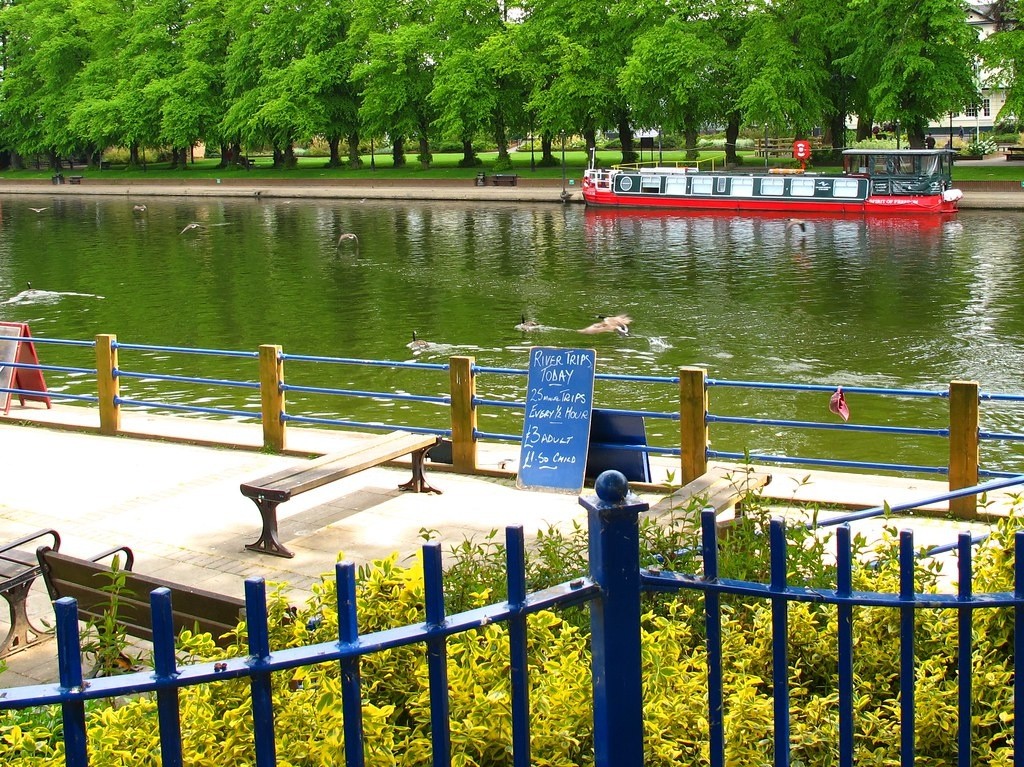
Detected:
[477, 172, 485, 186]
[56, 173, 64, 185]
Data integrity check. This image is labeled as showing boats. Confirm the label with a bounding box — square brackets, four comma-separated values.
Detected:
[574, 146, 964, 216]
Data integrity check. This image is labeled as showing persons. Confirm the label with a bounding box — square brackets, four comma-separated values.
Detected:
[958, 126, 964, 141]
[925, 133, 935, 149]
[945, 140, 951, 163]
[873, 122, 896, 140]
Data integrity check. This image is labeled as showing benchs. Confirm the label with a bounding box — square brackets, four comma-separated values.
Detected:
[69, 176, 83, 184]
[249, 160, 255, 167]
[491, 175, 520, 186]
[639, 465, 771, 558]
[102, 162, 111, 169]
[0, 527, 61, 658]
[35, 545, 302, 691]
[239, 431, 440, 558]
[909, 147, 961, 162]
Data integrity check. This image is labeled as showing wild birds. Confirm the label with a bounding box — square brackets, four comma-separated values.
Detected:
[180, 223, 209, 235]
[785, 218, 806, 232]
[337, 234, 359, 248]
[411, 330, 430, 347]
[576, 314, 634, 337]
[19, 282, 57, 298]
[134, 205, 147, 212]
[29, 207, 50, 213]
[520, 314, 541, 333]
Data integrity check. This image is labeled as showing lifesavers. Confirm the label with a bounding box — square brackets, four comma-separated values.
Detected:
[581, 176, 595, 192]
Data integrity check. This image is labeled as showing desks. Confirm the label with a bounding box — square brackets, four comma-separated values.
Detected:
[1003, 147, 1024, 162]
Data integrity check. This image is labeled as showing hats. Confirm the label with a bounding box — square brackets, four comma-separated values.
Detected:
[829, 385, 850, 422]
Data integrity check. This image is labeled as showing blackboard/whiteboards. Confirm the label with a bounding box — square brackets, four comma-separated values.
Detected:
[516, 346, 596, 494]
[0, 322, 49, 411]
